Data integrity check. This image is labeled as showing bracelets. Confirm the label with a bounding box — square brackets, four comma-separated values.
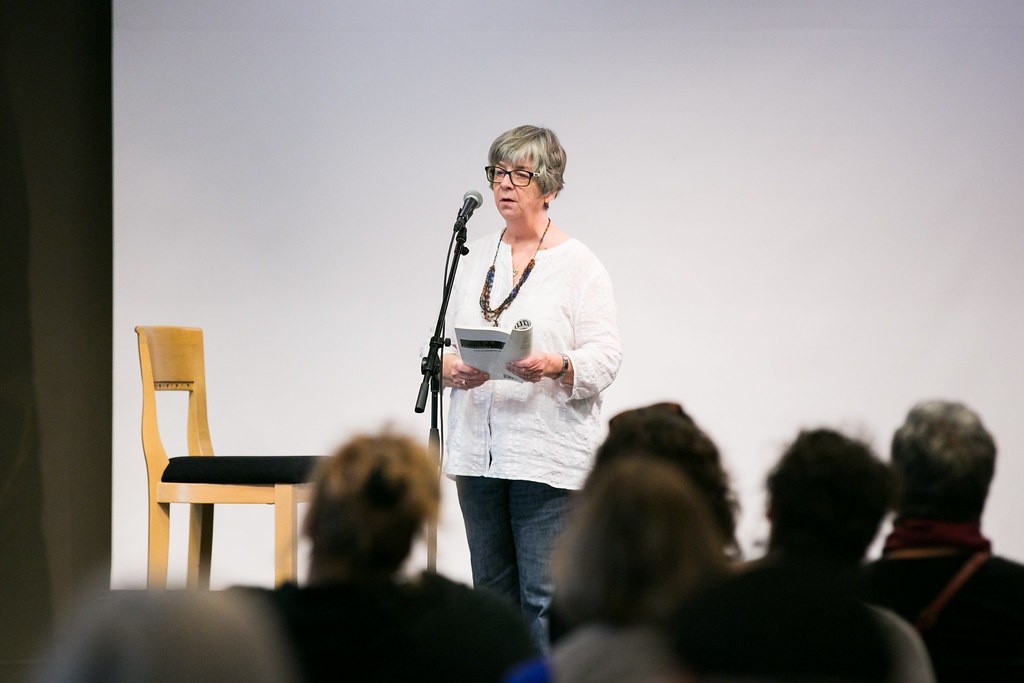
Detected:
[552, 353, 569, 381]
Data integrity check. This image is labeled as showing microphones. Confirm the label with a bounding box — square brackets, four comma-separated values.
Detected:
[454, 190, 483, 232]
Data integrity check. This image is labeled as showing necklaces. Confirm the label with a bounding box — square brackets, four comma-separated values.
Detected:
[480, 217, 550, 327]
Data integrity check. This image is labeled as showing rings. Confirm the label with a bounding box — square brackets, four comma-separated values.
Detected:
[528, 374, 531, 378]
[461, 380, 467, 385]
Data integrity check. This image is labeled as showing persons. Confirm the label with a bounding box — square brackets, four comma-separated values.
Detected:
[419, 124, 623, 658]
[40, 400, 1023, 683]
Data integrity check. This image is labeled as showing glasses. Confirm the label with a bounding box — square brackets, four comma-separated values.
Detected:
[485, 166, 540, 187]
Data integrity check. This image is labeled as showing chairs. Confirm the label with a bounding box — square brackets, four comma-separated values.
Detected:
[136, 325, 330, 591]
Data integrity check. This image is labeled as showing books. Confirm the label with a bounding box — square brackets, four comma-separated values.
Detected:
[453, 318, 534, 384]
